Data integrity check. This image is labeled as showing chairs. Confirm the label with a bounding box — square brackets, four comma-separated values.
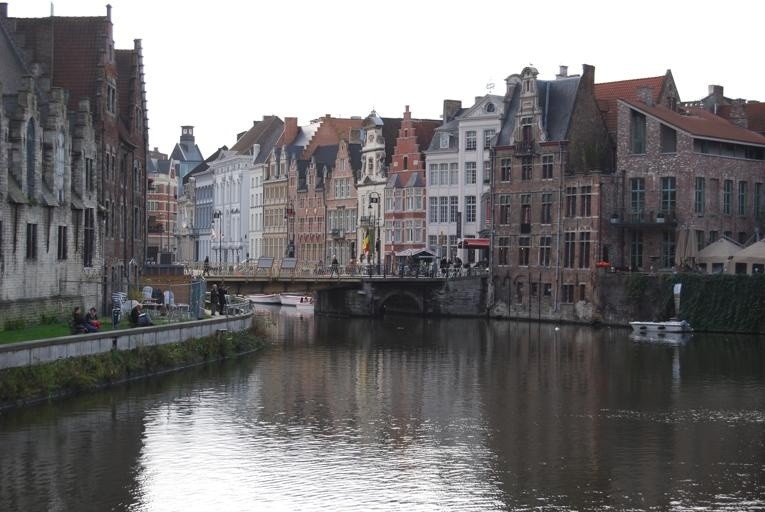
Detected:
[64, 286, 190, 335]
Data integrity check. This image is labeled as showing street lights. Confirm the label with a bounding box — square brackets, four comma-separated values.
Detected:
[212, 209, 223, 270]
[283, 199, 296, 273]
[367, 190, 383, 275]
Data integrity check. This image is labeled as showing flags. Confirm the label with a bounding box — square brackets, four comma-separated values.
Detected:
[361, 226, 375, 262]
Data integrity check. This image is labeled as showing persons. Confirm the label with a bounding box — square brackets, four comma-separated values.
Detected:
[210, 283, 219, 316]
[72, 306, 88, 334]
[318, 257, 324, 274]
[348, 255, 356, 276]
[130, 302, 153, 326]
[329, 255, 339, 278]
[202, 255, 211, 277]
[85, 307, 99, 332]
[218, 283, 231, 314]
[441, 253, 488, 279]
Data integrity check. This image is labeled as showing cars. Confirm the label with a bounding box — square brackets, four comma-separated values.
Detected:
[308, 258, 488, 279]
[605, 258, 703, 274]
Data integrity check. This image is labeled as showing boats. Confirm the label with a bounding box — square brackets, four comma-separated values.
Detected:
[250, 301, 313, 320]
[625, 317, 695, 334]
[231, 293, 313, 305]
[628, 331, 693, 346]
